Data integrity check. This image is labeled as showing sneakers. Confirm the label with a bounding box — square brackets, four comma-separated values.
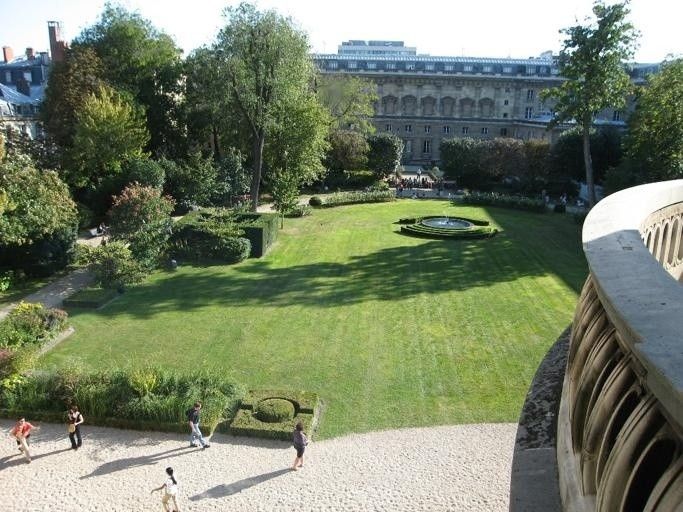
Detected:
[204, 445, 210, 448]
[190, 444, 197, 447]
[18, 447, 23, 452]
[172, 510, 180, 512]
[26, 457, 32, 463]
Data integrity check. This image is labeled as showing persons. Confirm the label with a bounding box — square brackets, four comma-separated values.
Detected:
[561, 192, 567, 207]
[291, 421, 309, 473]
[66, 405, 84, 449]
[150, 465, 181, 511]
[399, 177, 448, 190]
[544, 194, 550, 206]
[559, 191, 564, 204]
[8, 416, 40, 461]
[100, 222, 109, 232]
[95, 224, 105, 234]
[187, 401, 211, 448]
[416, 168, 421, 180]
